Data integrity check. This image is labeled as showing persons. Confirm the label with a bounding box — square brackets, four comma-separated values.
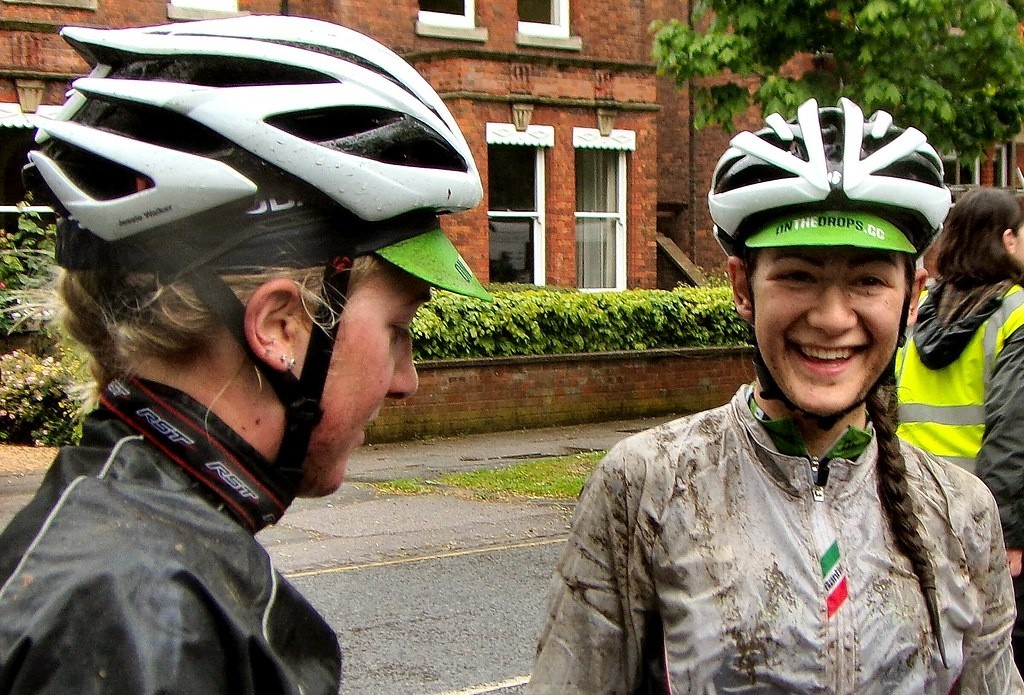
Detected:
[525, 94, 1024, 695]
[890, 183, 1022, 678]
[0, 14, 501, 695]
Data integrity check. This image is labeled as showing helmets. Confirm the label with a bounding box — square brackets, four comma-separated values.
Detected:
[22, 12, 500, 303]
[705, 96, 951, 265]
